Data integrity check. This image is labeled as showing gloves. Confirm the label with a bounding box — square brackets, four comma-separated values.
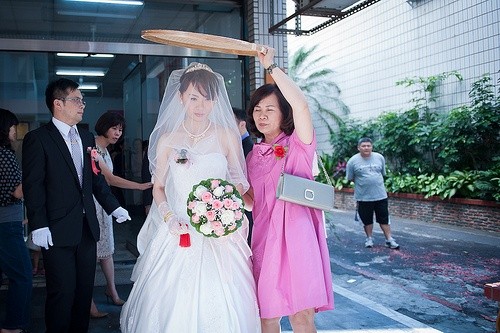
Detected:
[111, 206, 131, 224]
[31, 227, 54, 250]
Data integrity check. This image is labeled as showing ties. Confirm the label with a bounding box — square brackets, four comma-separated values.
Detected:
[69, 127, 83, 189]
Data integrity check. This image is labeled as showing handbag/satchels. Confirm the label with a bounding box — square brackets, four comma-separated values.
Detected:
[276, 138, 334, 212]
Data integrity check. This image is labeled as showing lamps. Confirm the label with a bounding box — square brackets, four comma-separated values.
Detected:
[54, 66, 107, 76]
[77, 82, 101, 90]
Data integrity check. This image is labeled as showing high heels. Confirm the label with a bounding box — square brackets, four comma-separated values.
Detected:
[104, 290, 125, 306]
[91, 311, 108, 317]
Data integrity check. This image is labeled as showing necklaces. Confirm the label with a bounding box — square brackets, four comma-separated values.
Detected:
[182, 119, 212, 142]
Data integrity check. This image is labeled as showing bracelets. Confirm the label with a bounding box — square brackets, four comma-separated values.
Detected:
[264, 63, 279, 75]
[163, 210, 175, 223]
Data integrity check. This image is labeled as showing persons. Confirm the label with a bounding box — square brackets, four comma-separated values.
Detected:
[231, 107, 254, 250]
[21, 77, 131, 333]
[89, 111, 152, 318]
[0, 108, 38, 333]
[245, 41, 336, 333]
[141, 145, 154, 214]
[345, 136, 400, 250]
[120, 61, 262, 333]
[130, 138, 144, 205]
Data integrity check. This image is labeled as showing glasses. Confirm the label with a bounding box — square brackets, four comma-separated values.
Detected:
[58, 97, 86, 106]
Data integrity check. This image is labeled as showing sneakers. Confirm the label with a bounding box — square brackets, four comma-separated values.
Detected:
[365, 237, 374, 248]
[385, 239, 400, 249]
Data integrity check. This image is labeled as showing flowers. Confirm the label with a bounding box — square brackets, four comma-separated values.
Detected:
[186, 178, 246, 239]
[87, 147, 100, 176]
[179, 224, 192, 247]
[273, 144, 289, 161]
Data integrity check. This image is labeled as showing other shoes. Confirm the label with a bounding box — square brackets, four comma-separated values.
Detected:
[42, 269, 45, 275]
[33, 271, 38, 274]
